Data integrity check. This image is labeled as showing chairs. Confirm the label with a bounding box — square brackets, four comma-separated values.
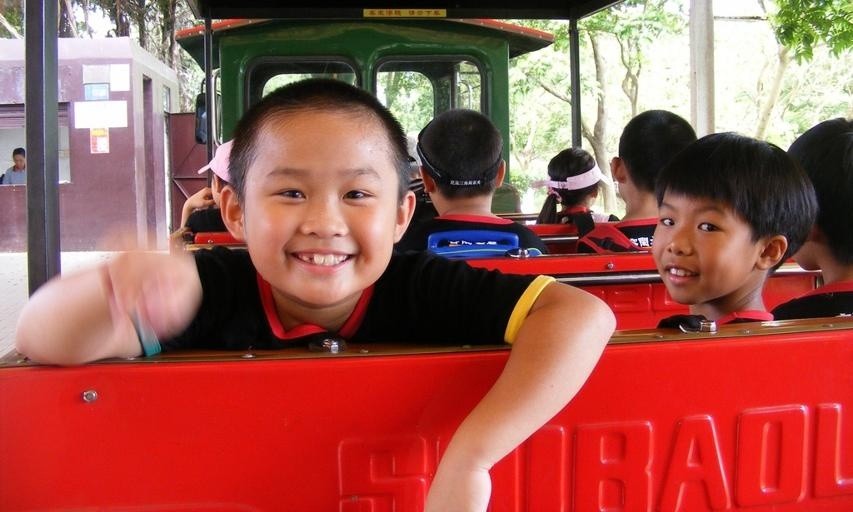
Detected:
[427, 229, 520, 260]
[191, 231, 243, 244]
[526, 222, 618, 254]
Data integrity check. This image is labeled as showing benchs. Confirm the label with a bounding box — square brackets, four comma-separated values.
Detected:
[1, 316, 853, 512]
[443, 248, 824, 330]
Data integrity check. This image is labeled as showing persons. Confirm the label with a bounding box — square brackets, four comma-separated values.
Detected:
[574, 107, 699, 251]
[177, 135, 237, 233]
[650, 129, 820, 331]
[10, 75, 618, 508]
[536, 146, 620, 225]
[396, 107, 549, 262]
[771, 115, 853, 320]
[2, 146, 26, 185]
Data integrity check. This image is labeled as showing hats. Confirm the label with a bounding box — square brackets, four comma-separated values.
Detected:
[547, 159, 611, 190]
[197, 138, 234, 185]
